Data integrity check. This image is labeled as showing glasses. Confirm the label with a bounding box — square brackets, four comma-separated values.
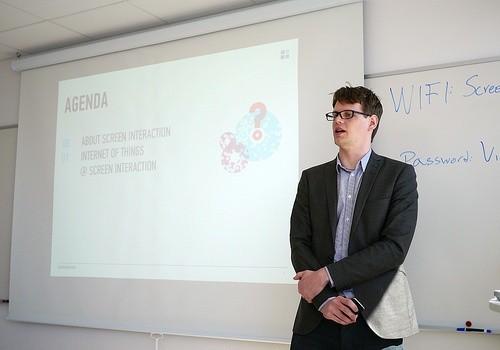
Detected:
[325, 110, 372, 121]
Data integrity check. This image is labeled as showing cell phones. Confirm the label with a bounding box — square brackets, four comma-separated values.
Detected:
[341, 297, 365, 318]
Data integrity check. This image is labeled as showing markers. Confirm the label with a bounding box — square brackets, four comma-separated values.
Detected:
[457, 327, 491, 333]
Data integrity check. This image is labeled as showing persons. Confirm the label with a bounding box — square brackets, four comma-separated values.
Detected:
[289, 81, 420, 350]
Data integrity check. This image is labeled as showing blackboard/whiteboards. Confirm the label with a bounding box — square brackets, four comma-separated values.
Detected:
[363, 57, 500, 335]
